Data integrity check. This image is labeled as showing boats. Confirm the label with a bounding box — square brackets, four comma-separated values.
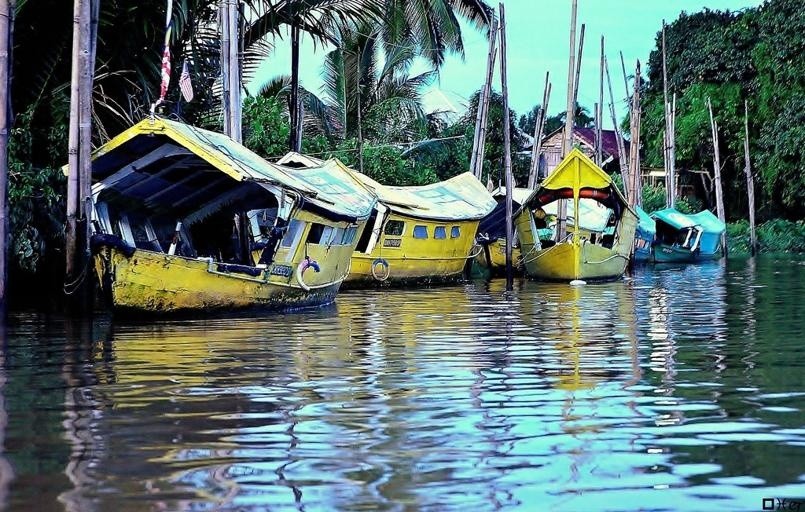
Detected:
[60, 116, 378, 315]
[277, 150, 499, 291]
[474, 148, 730, 282]
